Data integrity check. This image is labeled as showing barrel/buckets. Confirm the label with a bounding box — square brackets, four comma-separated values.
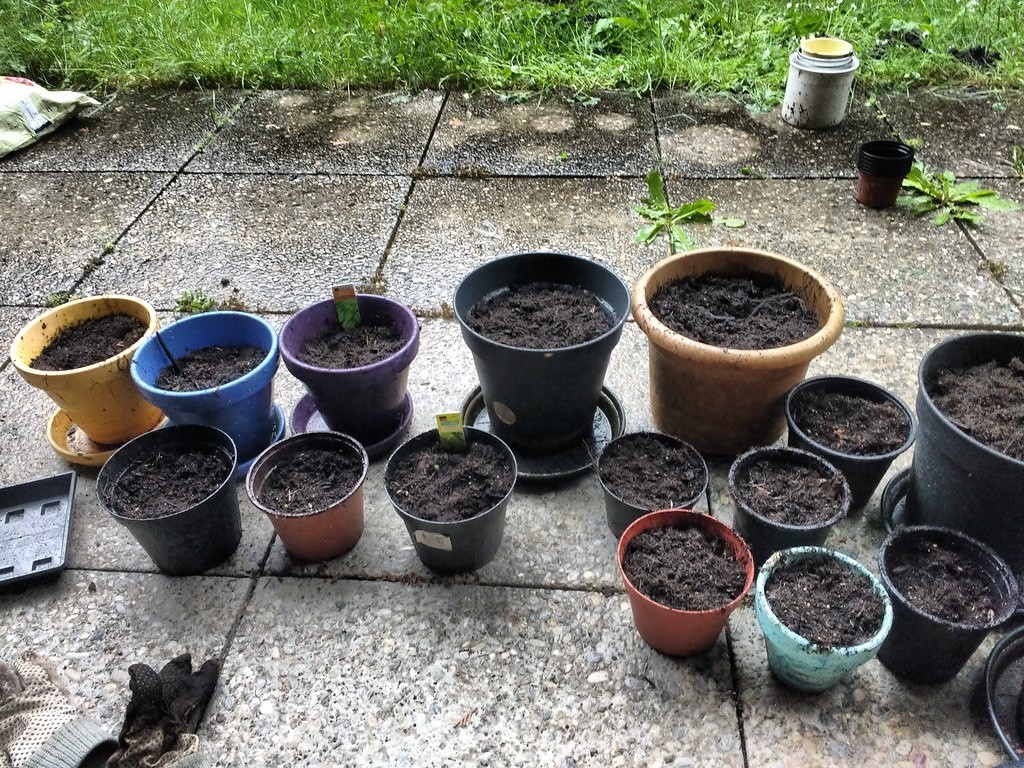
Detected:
[856, 138, 914, 207]
[782, 38, 859, 128]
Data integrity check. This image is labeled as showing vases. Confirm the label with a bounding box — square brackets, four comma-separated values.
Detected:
[625, 246, 847, 457]
[279, 286, 420, 443]
[595, 431, 707, 539]
[853, 140, 916, 212]
[131, 312, 281, 457]
[911, 333, 1024, 572]
[782, 39, 859, 129]
[243, 431, 369, 564]
[617, 508, 755, 656]
[729, 446, 851, 568]
[755, 545, 892, 693]
[879, 525, 1018, 686]
[6, 292, 159, 444]
[94, 423, 241, 576]
[786, 376, 915, 519]
[453, 249, 630, 462]
[385, 411, 518, 578]
